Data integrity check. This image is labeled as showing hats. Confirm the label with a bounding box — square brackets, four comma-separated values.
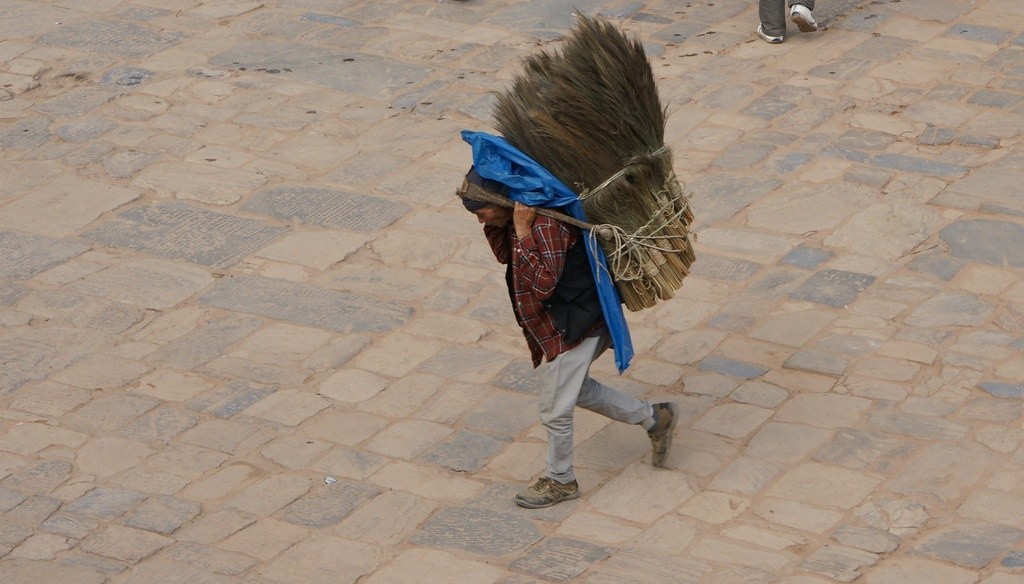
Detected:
[461, 167, 505, 210]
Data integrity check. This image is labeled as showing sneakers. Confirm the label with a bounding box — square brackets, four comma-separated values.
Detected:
[515, 475, 579, 509]
[757, 23, 783, 44]
[647, 402, 678, 467]
[790, 4, 817, 32]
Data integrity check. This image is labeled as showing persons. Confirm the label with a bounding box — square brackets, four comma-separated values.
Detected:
[457, 163, 679, 508]
[756, 1, 818, 44]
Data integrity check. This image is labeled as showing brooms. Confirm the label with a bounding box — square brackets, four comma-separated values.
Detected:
[485, 4, 698, 317]
